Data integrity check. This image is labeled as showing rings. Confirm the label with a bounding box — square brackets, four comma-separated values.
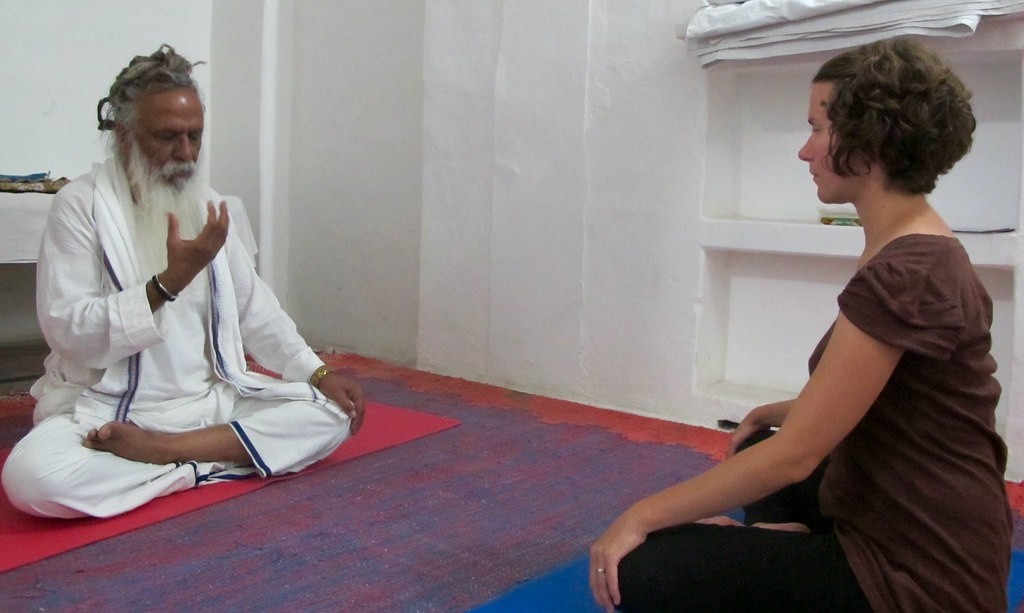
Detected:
[596, 569, 606, 574]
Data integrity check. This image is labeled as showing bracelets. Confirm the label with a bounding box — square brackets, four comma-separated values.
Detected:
[151, 274, 178, 302]
[309, 367, 335, 389]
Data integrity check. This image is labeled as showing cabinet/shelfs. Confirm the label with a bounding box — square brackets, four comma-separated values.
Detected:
[699, 0, 1024, 488]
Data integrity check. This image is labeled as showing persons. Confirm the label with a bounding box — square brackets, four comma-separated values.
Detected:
[590, 38, 1016, 613]
[2, 44, 366, 519]
